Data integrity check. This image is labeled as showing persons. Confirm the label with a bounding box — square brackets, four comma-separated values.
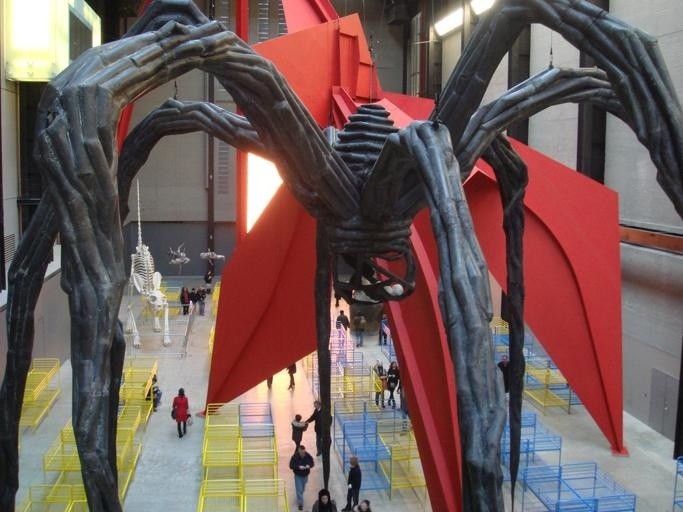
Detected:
[190, 287, 197, 306]
[179, 286, 190, 315]
[386, 361, 399, 410]
[284, 361, 296, 391]
[333, 290, 341, 308]
[351, 310, 367, 348]
[141, 374, 162, 413]
[172, 387, 188, 438]
[290, 414, 309, 448]
[266, 374, 273, 390]
[350, 499, 371, 512]
[288, 445, 314, 511]
[376, 311, 389, 346]
[340, 455, 362, 512]
[496, 354, 511, 394]
[196, 286, 207, 317]
[311, 488, 337, 512]
[335, 310, 349, 349]
[304, 400, 333, 457]
[372, 358, 386, 409]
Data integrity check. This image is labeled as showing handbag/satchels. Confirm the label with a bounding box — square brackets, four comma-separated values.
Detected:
[172, 409, 176, 419]
